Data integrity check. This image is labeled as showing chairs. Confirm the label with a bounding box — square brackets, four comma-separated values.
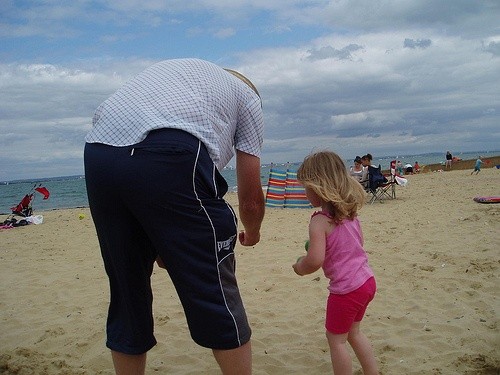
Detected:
[359, 160, 397, 203]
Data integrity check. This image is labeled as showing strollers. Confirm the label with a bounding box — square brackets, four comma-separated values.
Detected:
[12, 184, 42, 217]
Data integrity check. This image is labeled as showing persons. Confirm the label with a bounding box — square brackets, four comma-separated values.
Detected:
[292, 151, 379, 375]
[391, 161, 420, 180]
[83, 58, 265, 375]
[471, 155, 483, 175]
[349, 153, 378, 186]
[446, 151, 452, 170]
